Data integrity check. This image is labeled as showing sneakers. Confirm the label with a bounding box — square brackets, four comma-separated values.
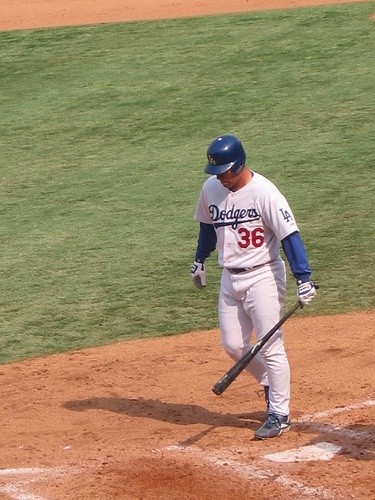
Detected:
[254, 414, 291, 439]
[257, 386, 269, 407]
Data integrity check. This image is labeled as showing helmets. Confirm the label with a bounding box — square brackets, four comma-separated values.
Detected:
[205, 136, 246, 174]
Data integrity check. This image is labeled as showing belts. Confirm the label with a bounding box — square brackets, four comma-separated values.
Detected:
[228, 263, 264, 274]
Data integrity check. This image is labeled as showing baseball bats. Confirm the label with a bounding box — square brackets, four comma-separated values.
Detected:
[211, 282, 320, 396]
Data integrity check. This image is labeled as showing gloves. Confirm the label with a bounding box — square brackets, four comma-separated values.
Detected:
[191, 257, 207, 289]
[296, 277, 316, 309]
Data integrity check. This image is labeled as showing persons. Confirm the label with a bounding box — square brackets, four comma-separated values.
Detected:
[191, 134, 318, 440]
